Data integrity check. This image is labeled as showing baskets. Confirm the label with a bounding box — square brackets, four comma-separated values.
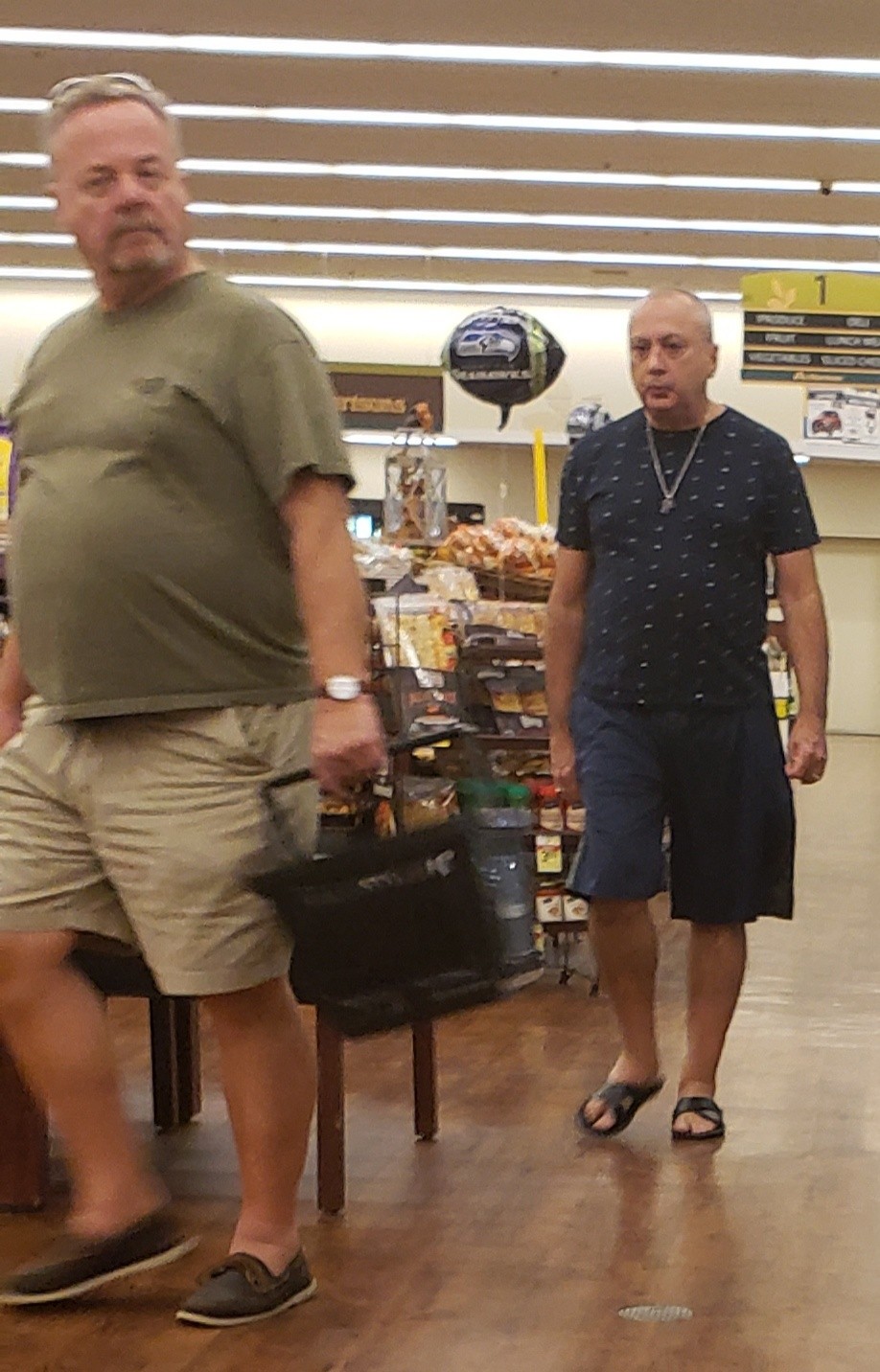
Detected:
[248, 725, 548, 1036]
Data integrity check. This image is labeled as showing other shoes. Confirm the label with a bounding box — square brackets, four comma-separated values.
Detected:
[177, 1247, 317, 1328]
[0, 1197, 204, 1307]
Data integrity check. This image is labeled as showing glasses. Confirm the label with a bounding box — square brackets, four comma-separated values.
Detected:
[44, 73, 155, 92]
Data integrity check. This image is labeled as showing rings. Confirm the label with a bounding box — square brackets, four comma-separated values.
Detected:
[812, 773, 820, 780]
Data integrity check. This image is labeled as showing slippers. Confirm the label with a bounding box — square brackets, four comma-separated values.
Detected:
[571, 1073, 667, 1140]
[668, 1096, 728, 1141]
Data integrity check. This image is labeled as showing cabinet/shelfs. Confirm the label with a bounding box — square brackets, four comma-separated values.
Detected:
[774, 460, 880, 733]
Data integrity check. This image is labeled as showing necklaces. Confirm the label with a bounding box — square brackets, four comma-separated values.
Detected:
[646, 402, 714, 515]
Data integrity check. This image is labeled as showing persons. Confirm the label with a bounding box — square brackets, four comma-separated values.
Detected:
[545, 287, 831, 1142]
[1, 73, 382, 1328]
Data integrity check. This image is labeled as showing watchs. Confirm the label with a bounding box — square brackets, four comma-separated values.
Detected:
[308, 676, 378, 702]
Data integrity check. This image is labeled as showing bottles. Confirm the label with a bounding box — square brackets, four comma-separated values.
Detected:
[460, 777, 587, 833]
